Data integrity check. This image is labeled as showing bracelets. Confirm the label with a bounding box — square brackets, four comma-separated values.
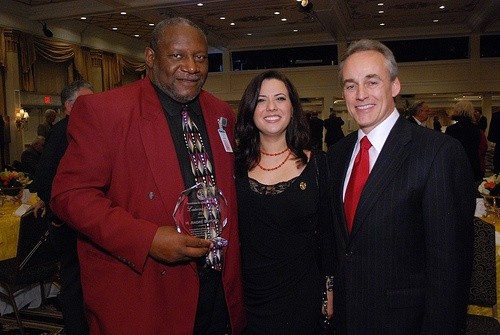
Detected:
[323, 275, 335, 293]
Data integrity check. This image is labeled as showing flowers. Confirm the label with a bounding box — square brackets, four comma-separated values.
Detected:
[0, 168, 33, 186]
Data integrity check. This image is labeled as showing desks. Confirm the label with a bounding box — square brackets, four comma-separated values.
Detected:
[0, 192, 41, 261]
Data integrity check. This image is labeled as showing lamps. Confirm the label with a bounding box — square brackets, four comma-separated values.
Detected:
[40, 22, 54, 38]
[16, 109, 30, 133]
[296, 0, 313, 14]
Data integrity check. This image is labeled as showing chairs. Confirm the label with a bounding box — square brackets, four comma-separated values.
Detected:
[466, 217, 500, 335]
[0, 208, 59, 335]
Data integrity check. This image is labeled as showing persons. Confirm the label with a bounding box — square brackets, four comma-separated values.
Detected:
[48, 16, 248, 335]
[37, 108, 57, 136]
[323, 113, 345, 148]
[444, 101, 481, 184]
[476, 115, 488, 178]
[487, 111, 500, 175]
[406, 99, 429, 129]
[325, 38, 479, 335]
[30, 78, 98, 335]
[308, 111, 323, 151]
[20, 135, 46, 180]
[230, 67, 335, 335]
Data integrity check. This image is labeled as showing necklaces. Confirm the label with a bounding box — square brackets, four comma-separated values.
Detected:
[252, 142, 290, 174]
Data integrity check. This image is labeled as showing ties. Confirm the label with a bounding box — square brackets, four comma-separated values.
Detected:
[343, 135, 373, 236]
[180, 104, 223, 271]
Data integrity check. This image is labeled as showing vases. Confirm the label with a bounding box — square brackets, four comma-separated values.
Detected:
[2, 187, 20, 196]
